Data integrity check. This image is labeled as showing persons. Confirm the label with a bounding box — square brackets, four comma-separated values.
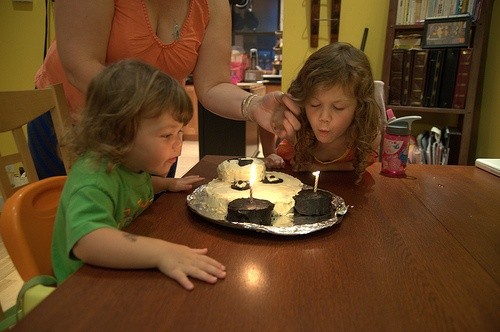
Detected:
[27, 1, 302, 181]
[51, 60, 226, 290]
[263, 41, 384, 187]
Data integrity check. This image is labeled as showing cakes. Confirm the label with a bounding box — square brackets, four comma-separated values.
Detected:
[204, 156, 334, 226]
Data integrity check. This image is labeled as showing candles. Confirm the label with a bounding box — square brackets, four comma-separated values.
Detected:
[312, 170, 320, 192]
[248, 162, 258, 205]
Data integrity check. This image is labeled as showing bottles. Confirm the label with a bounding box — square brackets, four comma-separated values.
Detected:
[380, 108, 422, 177]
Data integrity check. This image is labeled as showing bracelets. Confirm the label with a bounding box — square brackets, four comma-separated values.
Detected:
[240, 94, 258, 122]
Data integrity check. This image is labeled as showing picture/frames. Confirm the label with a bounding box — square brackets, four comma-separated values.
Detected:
[421, 16, 470, 49]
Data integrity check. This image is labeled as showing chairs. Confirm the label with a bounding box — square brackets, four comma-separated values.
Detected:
[0, 81, 73, 200]
[0, 176, 67, 281]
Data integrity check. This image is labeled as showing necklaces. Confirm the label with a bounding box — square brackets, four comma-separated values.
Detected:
[167, 0, 186, 41]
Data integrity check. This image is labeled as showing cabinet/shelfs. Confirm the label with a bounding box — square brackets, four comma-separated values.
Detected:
[381, 0, 495, 166]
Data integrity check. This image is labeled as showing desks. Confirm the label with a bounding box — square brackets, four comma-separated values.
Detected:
[12, 155, 500, 332]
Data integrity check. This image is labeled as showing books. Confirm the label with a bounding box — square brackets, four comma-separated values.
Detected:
[387, 33, 473, 110]
[396, 0, 478, 26]
[419, 128, 462, 166]
